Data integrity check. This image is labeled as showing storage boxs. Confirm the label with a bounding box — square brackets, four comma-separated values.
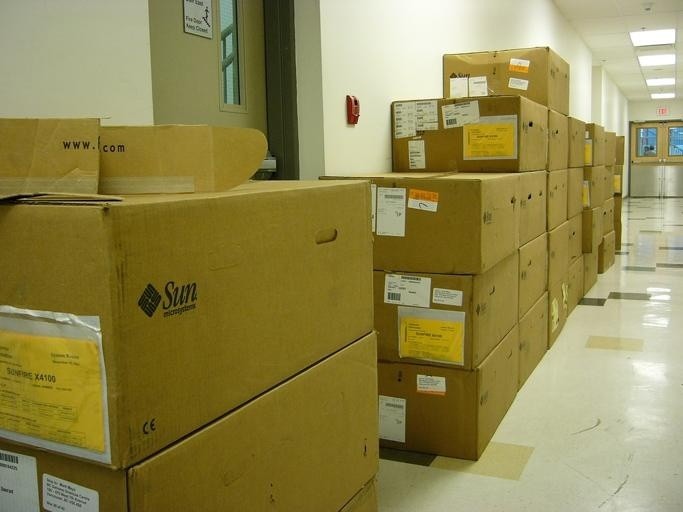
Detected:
[318, 48, 626, 463]
[0, 108, 382, 510]
